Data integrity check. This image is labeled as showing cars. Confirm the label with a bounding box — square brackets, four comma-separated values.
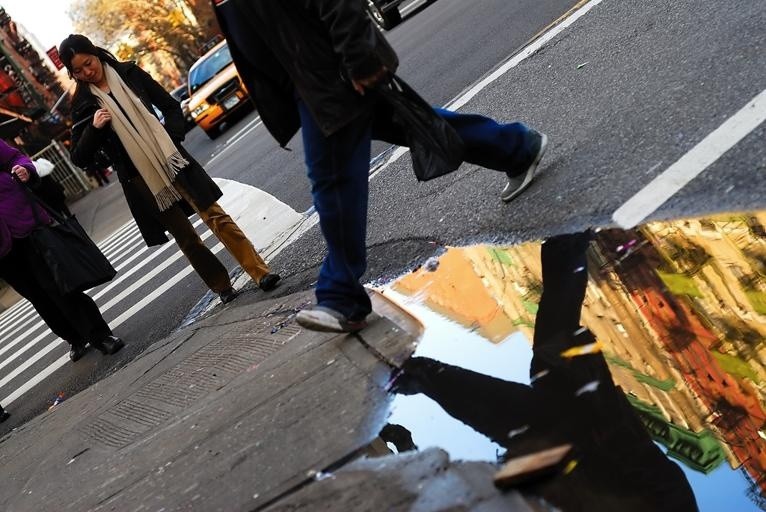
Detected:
[153, 34, 253, 140]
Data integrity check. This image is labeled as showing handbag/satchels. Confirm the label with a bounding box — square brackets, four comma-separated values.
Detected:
[10, 170, 118, 294]
[71, 113, 112, 177]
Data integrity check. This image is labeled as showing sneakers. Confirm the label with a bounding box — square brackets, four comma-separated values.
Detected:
[500, 131, 548, 202]
[295, 307, 368, 333]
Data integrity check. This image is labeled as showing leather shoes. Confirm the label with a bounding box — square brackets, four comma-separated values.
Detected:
[219, 286, 239, 304]
[259, 273, 280, 292]
[70, 335, 125, 362]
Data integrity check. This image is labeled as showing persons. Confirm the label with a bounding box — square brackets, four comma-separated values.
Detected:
[1, 141, 124, 363]
[0, 404, 10, 423]
[212, 1, 549, 337]
[389, 233, 699, 511]
[83, 150, 111, 188]
[58, 34, 283, 305]
